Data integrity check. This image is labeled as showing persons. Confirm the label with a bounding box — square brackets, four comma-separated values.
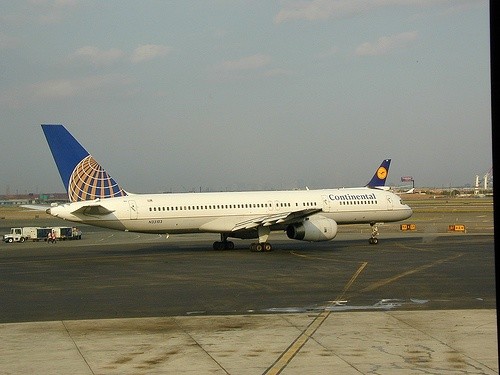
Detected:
[48, 232, 56, 243]
[66, 228, 82, 240]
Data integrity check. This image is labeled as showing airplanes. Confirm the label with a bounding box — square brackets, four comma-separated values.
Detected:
[19, 124, 412, 253]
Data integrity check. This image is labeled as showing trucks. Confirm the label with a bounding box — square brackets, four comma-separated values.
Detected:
[2, 226, 39, 243]
